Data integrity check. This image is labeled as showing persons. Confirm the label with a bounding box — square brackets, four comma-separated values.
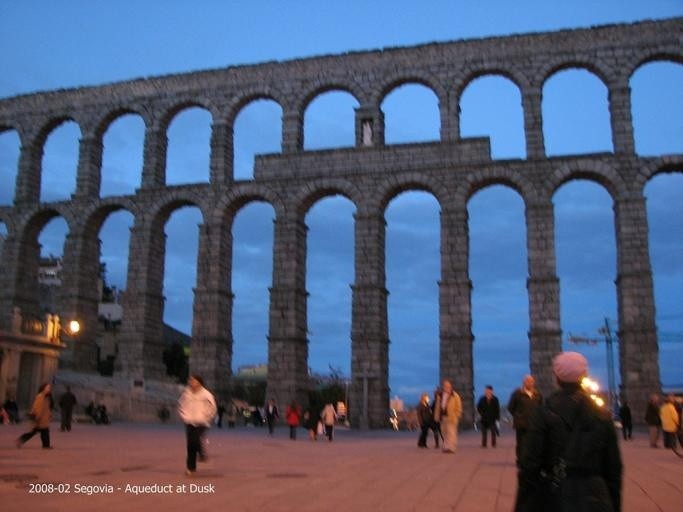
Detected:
[514, 352, 622, 510]
[152, 372, 349, 443]
[180, 374, 217, 473]
[621, 391, 683, 449]
[413, 375, 543, 454]
[0, 381, 109, 449]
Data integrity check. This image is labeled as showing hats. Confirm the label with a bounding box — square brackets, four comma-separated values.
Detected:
[551, 350, 589, 383]
